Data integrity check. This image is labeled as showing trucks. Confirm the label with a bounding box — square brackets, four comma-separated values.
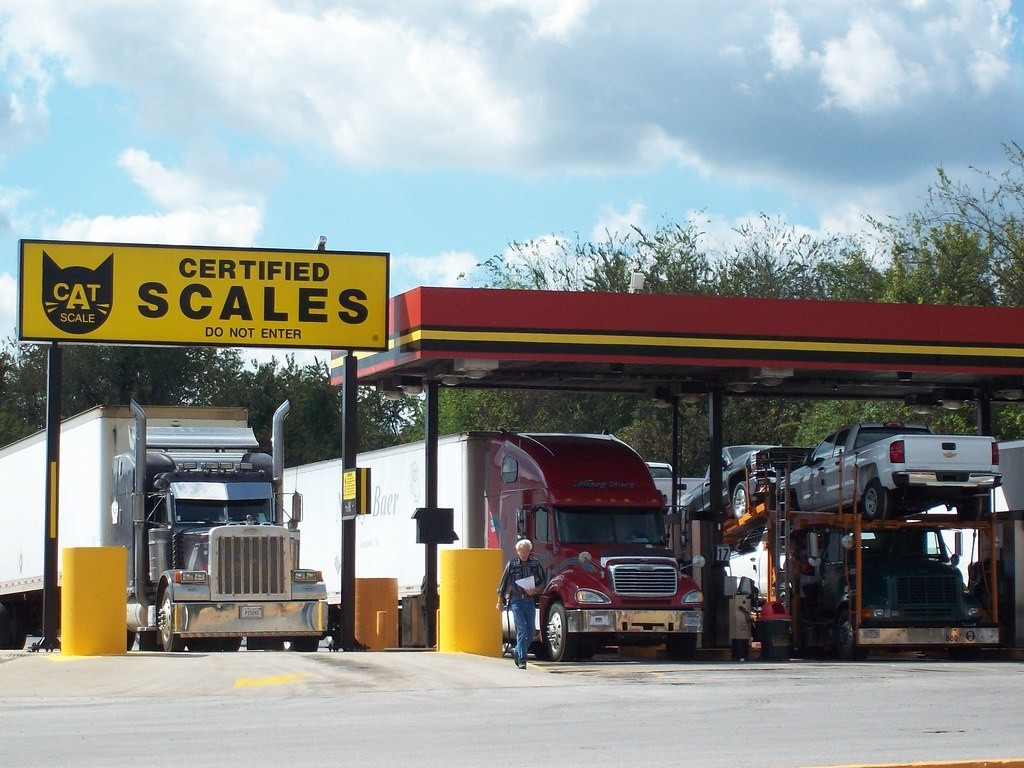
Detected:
[655, 477, 1000, 661]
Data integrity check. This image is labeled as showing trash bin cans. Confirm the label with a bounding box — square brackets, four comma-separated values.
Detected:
[756, 601, 793, 663]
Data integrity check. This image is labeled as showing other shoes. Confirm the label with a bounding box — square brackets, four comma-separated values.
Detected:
[519, 662, 526, 669]
[511, 648, 519, 667]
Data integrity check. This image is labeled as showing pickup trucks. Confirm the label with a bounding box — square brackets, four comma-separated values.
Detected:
[774, 422, 1003, 521]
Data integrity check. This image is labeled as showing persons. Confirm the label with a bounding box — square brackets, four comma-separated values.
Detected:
[949, 555, 959, 567]
[496, 538, 546, 669]
[970, 562, 987, 609]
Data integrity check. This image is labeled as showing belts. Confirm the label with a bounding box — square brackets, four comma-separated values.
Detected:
[512, 593, 533, 600]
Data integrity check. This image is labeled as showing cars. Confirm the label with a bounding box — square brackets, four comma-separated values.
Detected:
[669, 442, 822, 522]
[723, 531, 787, 600]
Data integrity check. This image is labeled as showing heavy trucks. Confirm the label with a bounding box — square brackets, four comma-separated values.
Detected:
[0, 396, 329, 653]
[283, 428, 706, 663]
